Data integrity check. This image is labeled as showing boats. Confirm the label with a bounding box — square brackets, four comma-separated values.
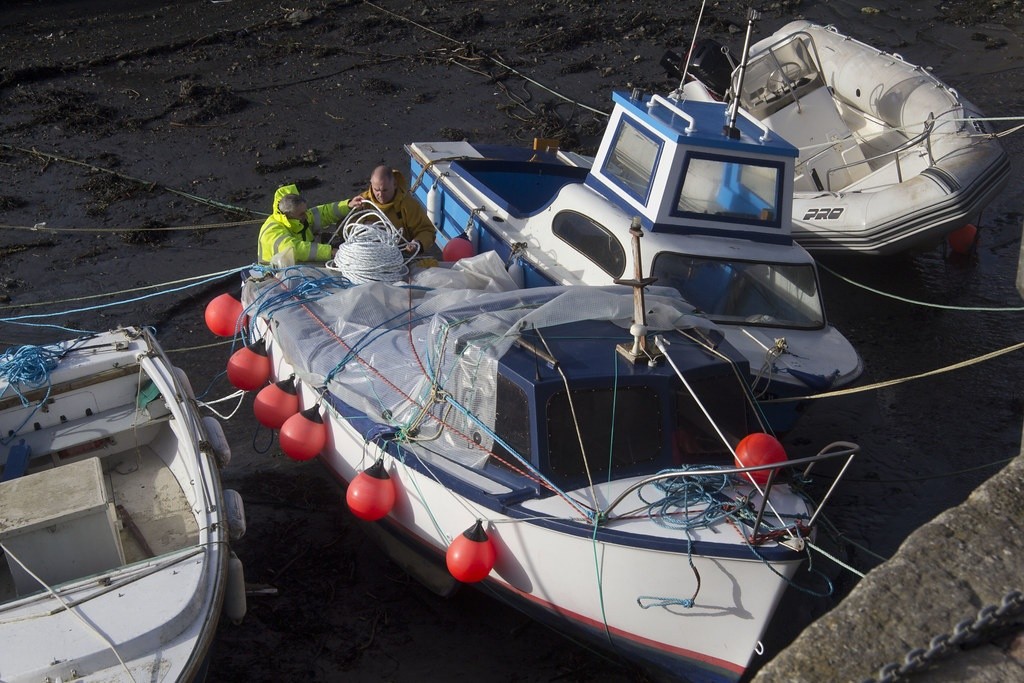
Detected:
[0, 323, 248, 683]
[402, 1, 866, 441]
[237, 248, 863, 682]
[669, 19, 1015, 268]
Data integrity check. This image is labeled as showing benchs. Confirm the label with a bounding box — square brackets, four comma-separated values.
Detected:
[826, 112, 935, 193]
[0, 404, 170, 467]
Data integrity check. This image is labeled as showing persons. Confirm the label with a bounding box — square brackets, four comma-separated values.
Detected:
[350, 165, 437, 255]
[258, 185, 364, 267]
